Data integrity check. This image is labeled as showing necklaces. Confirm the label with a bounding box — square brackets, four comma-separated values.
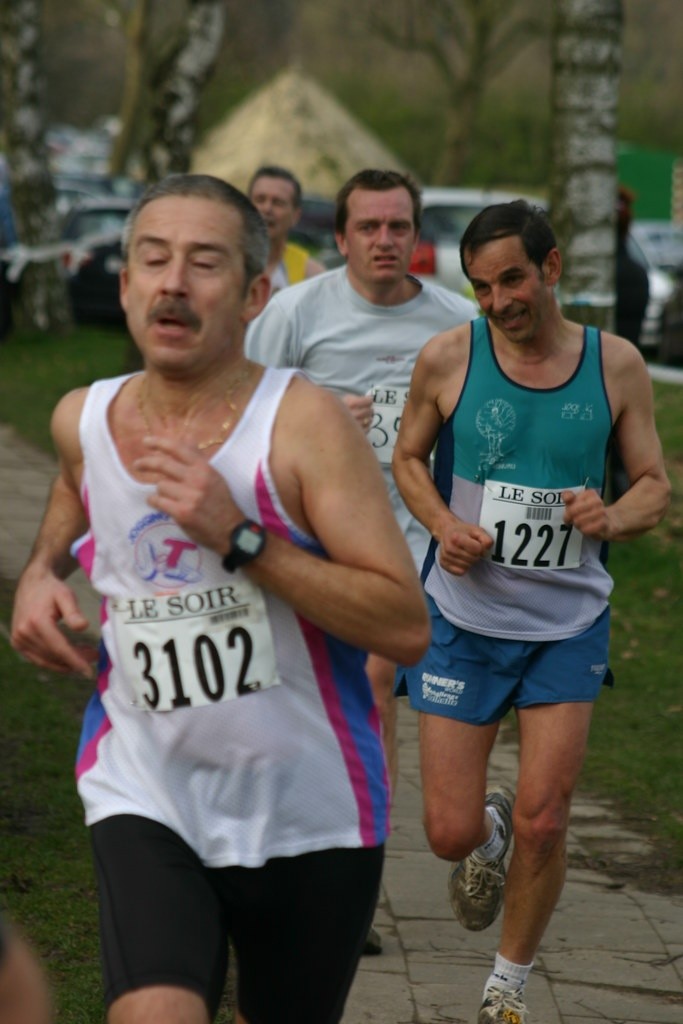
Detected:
[136, 364, 250, 456]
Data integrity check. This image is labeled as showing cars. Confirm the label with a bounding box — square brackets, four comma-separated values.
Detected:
[3, 171, 137, 332]
[297, 185, 683, 362]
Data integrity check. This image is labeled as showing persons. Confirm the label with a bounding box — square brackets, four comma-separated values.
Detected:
[389, 197, 673, 1024]
[244, 169, 481, 956]
[604, 189, 651, 688]
[11, 174, 431, 1024]
[248, 166, 328, 292]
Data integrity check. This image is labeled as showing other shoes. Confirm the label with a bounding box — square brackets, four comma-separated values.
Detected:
[363, 925, 383, 956]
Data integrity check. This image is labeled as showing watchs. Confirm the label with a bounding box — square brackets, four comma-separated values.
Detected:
[221, 519, 266, 574]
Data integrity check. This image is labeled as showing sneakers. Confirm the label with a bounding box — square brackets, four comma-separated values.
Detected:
[448, 785, 516, 932]
[477, 987, 530, 1024]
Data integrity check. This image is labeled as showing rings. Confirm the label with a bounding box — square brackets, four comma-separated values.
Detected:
[365, 419, 368, 426]
